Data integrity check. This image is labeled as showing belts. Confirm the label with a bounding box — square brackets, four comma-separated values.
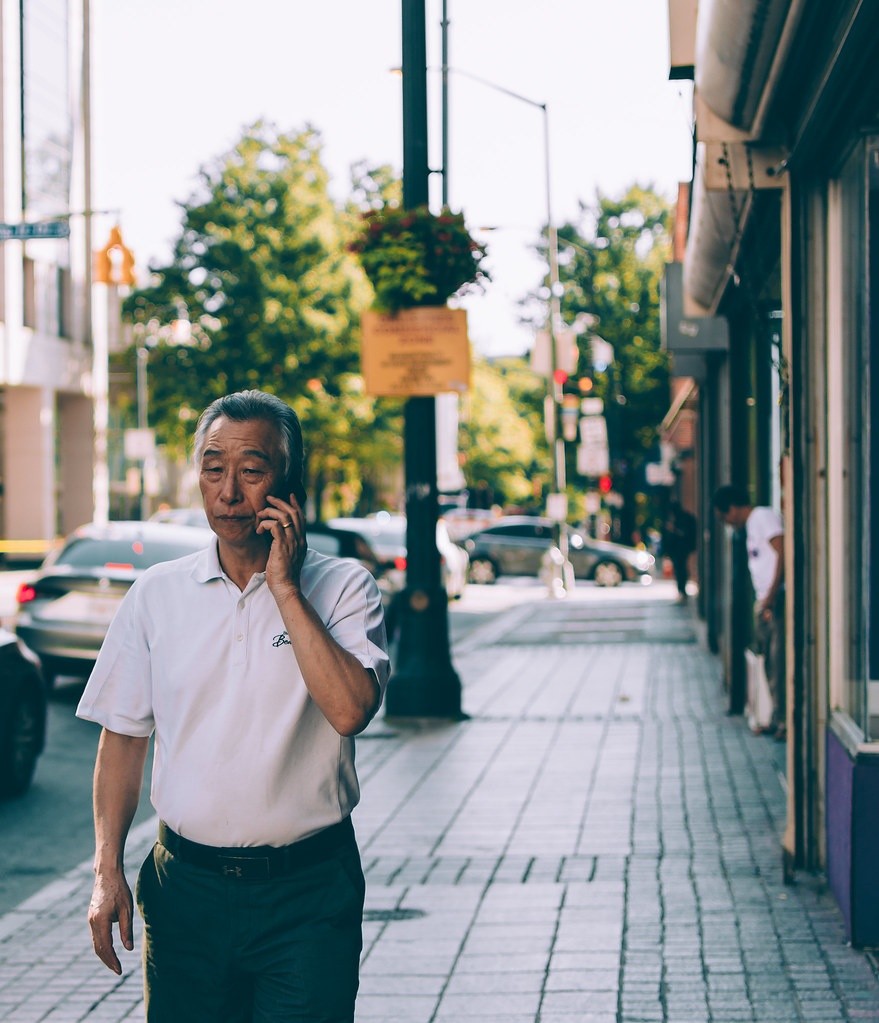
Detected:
[159, 819, 347, 879]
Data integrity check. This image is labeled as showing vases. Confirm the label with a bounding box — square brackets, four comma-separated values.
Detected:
[358, 306, 473, 395]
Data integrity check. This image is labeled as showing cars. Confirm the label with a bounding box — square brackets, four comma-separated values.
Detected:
[454, 516, 655, 591]
[13, 519, 219, 686]
[0, 629, 48, 801]
[302, 520, 396, 610]
[325, 510, 473, 603]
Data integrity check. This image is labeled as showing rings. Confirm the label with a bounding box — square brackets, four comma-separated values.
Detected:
[281, 522, 293, 528]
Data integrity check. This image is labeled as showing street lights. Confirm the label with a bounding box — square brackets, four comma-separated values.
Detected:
[389, 62, 575, 592]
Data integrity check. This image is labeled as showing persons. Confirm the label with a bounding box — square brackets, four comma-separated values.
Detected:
[664, 499, 697, 606]
[74, 390, 392, 1023]
[710, 485, 786, 742]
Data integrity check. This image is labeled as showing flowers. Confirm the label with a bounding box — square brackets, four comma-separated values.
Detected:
[343, 200, 499, 318]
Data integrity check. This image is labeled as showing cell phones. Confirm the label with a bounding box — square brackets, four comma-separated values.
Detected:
[261, 478, 307, 552]
